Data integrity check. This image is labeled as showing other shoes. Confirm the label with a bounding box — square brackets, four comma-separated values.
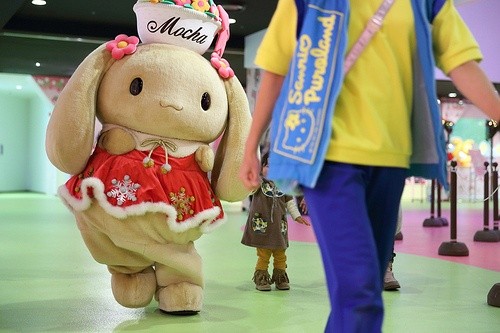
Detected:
[383, 261, 400, 290]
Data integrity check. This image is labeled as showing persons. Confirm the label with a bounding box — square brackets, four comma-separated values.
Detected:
[241, 152, 310, 290]
[384, 202, 403, 289]
[240, 1, 500, 333]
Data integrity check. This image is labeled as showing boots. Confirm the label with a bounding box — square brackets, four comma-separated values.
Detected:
[254, 270, 271, 291]
[273, 269, 290, 290]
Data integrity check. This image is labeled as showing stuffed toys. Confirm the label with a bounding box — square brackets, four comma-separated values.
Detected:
[45, 35, 260, 311]
